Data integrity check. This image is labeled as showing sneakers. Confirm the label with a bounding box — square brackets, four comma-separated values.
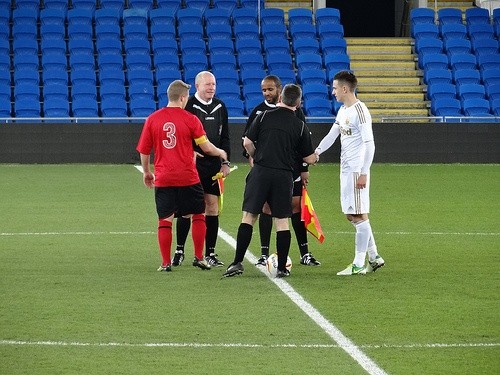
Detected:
[223, 261, 244, 278]
[170, 251, 184, 266]
[276, 268, 290, 277]
[255, 254, 269, 266]
[204, 254, 224, 267]
[336, 263, 366, 275]
[300, 252, 320, 266]
[193, 256, 211, 270]
[157, 265, 171, 271]
[365, 256, 385, 273]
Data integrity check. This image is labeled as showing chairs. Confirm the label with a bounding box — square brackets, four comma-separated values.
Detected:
[410, 8, 500, 123]
[0, 0, 357, 124]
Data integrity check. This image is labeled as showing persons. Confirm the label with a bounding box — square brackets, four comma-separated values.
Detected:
[136, 70, 321, 278]
[313, 71, 386, 276]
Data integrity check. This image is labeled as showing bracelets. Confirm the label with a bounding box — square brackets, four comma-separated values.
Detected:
[221, 161, 231, 166]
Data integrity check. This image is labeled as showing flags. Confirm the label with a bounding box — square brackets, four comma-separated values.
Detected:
[215, 172, 224, 215]
[301, 183, 324, 243]
